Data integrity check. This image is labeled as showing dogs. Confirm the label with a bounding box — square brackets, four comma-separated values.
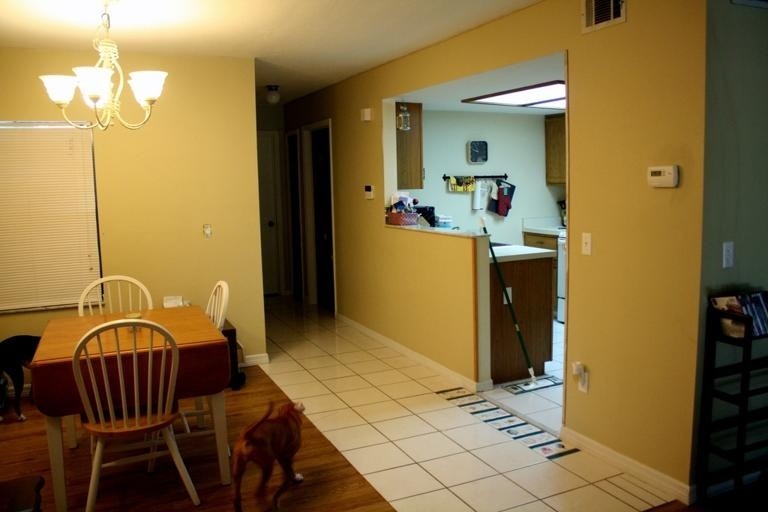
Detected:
[0, 335, 42, 422]
[231, 401, 305, 511]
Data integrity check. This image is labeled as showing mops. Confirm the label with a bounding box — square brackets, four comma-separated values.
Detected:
[478, 215, 563, 395]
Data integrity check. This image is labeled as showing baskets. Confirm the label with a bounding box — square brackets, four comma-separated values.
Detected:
[389, 210, 418, 226]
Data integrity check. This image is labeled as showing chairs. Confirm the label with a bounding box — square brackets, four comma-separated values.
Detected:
[147, 279, 229, 474]
[71, 317, 202, 512]
[78, 274, 153, 317]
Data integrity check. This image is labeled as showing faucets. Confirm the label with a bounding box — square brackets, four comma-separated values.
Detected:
[451, 224, 461, 232]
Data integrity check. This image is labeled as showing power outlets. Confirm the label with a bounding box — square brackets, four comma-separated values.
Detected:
[580, 373, 589, 392]
[723, 241, 734, 267]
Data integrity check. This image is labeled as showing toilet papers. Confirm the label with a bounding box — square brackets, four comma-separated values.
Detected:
[472, 180, 483, 211]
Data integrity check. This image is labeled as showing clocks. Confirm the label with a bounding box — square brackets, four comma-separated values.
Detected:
[467, 140, 488, 164]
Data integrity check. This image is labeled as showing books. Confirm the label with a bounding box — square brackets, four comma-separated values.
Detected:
[710, 289, 768, 338]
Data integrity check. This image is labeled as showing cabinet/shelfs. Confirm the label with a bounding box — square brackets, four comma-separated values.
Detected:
[521, 232, 557, 320]
[698, 282, 767, 512]
[397, 101, 424, 189]
[546, 113, 567, 184]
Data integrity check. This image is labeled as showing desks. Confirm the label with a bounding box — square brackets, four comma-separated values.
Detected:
[32, 305, 228, 512]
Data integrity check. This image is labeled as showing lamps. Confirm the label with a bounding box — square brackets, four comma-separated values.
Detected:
[41, 0, 166, 133]
[265, 84, 281, 104]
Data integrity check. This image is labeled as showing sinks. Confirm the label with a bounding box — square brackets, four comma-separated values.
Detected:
[490, 241, 510, 247]
